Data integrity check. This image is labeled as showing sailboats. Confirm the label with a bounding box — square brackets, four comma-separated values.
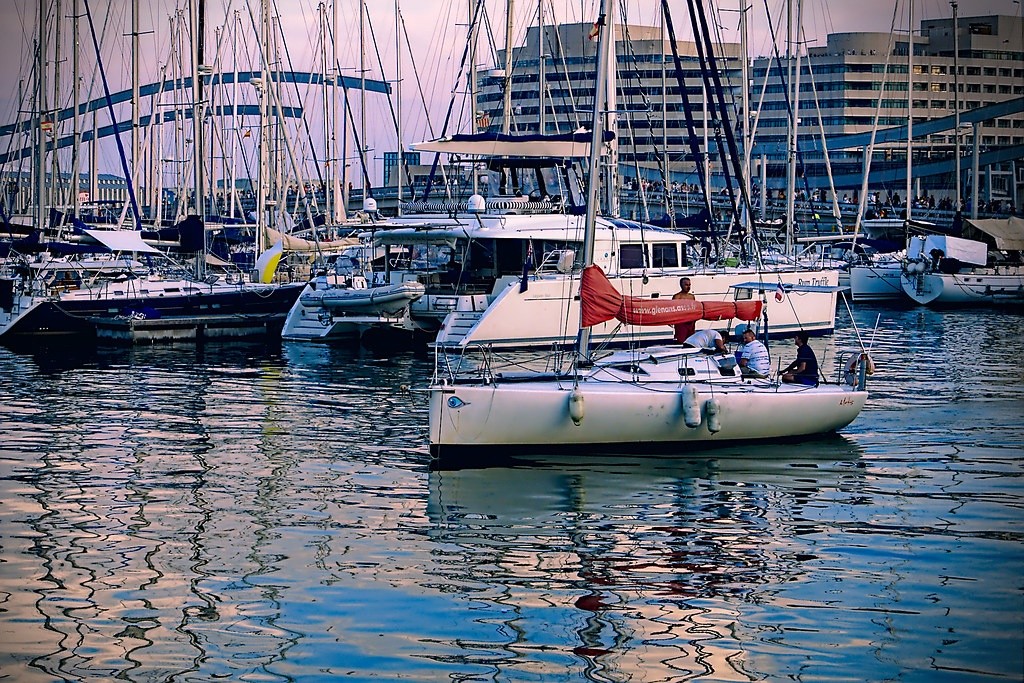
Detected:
[1, 1, 1024, 462]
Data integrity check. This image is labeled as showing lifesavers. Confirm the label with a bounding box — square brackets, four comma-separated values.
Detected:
[844, 352, 875, 386]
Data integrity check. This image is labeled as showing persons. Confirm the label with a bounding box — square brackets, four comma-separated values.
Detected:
[628, 177, 827, 207]
[843, 189, 1017, 219]
[739, 328, 770, 378]
[780, 333, 820, 386]
[683, 330, 729, 354]
[670, 277, 696, 342]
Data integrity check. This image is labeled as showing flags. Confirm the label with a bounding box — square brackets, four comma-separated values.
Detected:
[775, 282, 783, 301]
